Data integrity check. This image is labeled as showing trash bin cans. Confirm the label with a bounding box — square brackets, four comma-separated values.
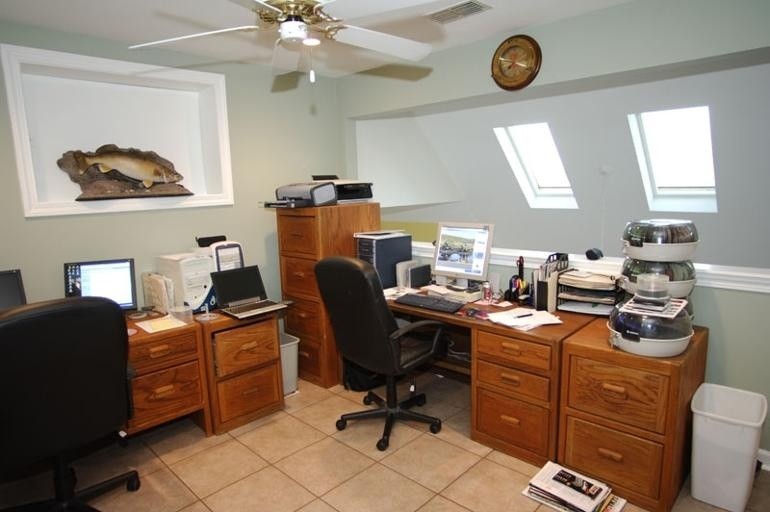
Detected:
[279, 332, 301, 397]
[690, 381, 768, 512]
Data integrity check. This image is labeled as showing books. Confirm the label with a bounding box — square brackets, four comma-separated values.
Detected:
[142, 269, 176, 316]
[618, 295, 689, 320]
[488, 267, 615, 333]
[520, 459, 627, 512]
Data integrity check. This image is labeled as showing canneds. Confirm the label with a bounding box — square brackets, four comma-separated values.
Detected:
[481, 281, 493, 301]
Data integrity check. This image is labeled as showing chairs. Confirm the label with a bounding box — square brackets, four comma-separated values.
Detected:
[0, 296, 143, 510]
[312, 255, 450, 450]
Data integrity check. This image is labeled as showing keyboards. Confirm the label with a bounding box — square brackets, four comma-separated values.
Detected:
[394, 294, 464, 314]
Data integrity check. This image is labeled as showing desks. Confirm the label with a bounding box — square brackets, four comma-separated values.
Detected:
[381, 285, 594, 469]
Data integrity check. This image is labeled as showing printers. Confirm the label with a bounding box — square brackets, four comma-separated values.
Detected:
[312, 175, 373, 200]
[263, 182, 336, 208]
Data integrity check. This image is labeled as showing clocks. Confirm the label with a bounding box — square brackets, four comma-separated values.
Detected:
[490, 33, 542, 91]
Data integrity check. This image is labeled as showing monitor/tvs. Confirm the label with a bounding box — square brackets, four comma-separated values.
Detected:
[431, 221, 495, 290]
[0, 269, 26, 313]
[64, 258, 136, 311]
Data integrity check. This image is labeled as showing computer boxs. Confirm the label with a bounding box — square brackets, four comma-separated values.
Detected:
[356, 233, 412, 289]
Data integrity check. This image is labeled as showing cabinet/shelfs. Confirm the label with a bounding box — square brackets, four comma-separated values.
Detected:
[123, 304, 213, 439]
[279, 200, 381, 391]
[196, 304, 285, 435]
[562, 314, 709, 511]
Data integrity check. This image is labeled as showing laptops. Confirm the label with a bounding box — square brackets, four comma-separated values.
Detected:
[211, 265, 288, 320]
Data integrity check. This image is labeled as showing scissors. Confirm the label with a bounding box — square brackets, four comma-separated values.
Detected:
[517, 256, 524, 267]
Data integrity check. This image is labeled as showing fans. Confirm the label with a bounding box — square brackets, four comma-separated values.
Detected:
[126, 1, 435, 85]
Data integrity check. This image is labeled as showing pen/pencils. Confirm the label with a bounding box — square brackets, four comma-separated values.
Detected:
[517, 314, 532, 318]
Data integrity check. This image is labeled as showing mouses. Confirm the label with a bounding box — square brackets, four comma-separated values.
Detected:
[467, 308, 477, 316]
